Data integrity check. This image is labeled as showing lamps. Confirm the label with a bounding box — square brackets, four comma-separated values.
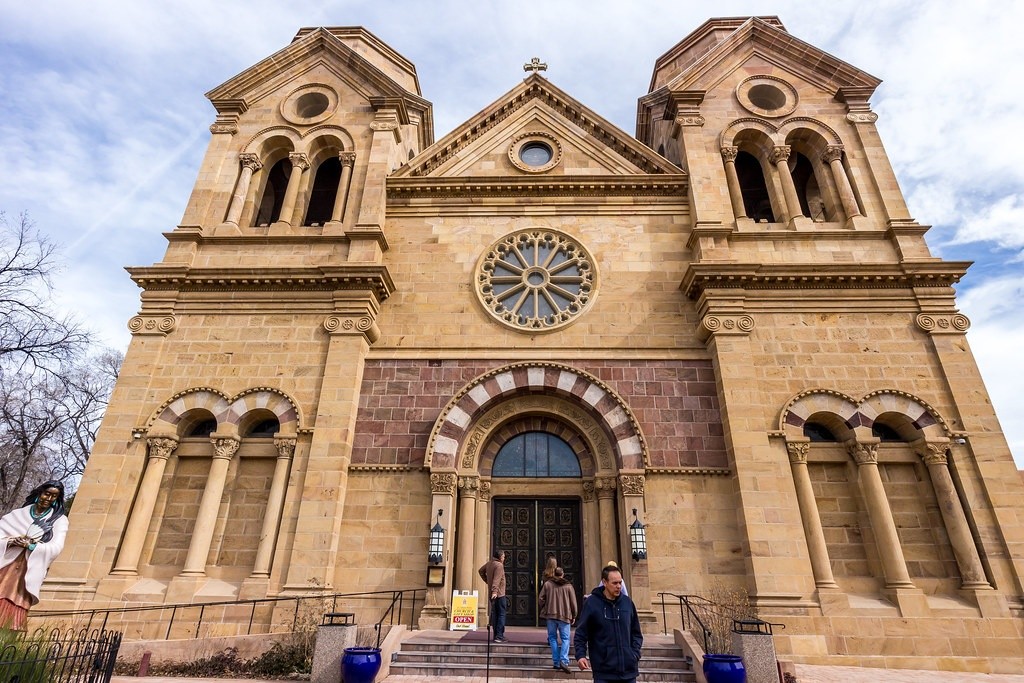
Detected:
[428, 509, 444, 565]
[628, 508, 647, 562]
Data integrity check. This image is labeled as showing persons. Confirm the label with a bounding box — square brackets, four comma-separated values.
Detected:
[478, 550, 509, 643]
[573, 566, 643, 683]
[542, 557, 557, 583]
[0, 480, 69, 632]
[539, 567, 578, 674]
[599, 561, 628, 597]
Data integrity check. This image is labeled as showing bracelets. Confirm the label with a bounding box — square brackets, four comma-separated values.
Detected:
[28, 544, 36, 551]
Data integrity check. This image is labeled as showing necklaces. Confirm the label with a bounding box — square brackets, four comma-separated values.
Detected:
[31, 505, 51, 519]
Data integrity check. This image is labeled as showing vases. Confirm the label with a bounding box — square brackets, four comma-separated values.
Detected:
[341, 646, 382, 683]
[702, 654, 746, 683]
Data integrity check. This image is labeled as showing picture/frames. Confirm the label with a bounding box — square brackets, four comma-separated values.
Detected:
[426, 565, 446, 587]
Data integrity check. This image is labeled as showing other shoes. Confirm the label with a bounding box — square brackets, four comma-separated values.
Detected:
[494, 636, 510, 643]
[548, 641, 552, 645]
[559, 661, 572, 674]
[554, 665, 561, 669]
